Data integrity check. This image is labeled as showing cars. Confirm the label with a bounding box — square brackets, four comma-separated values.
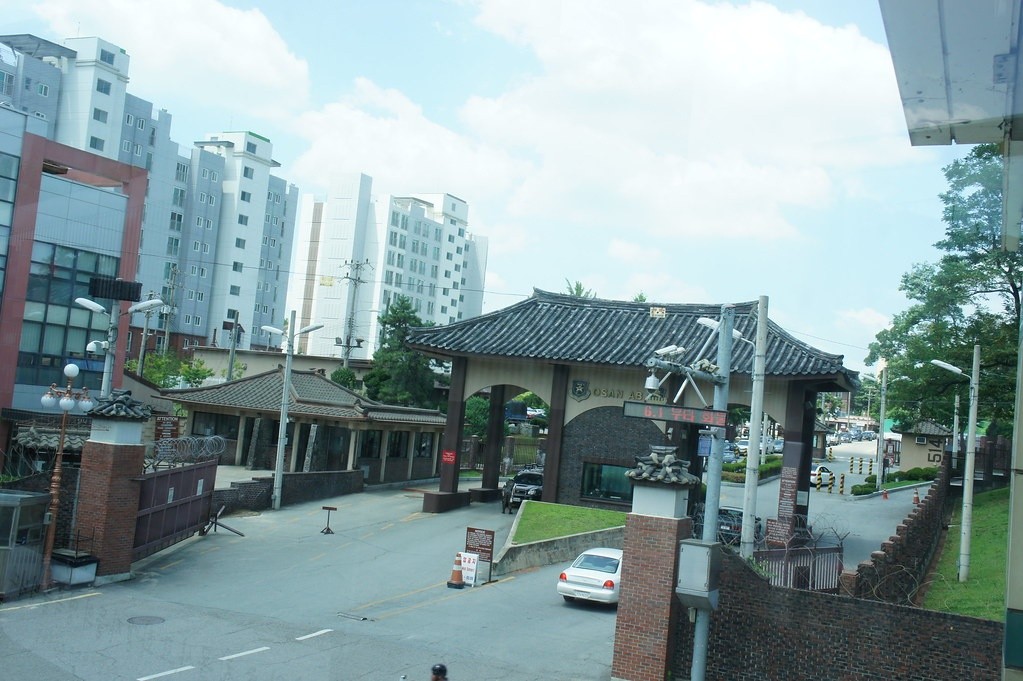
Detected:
[826, 429, 877, 446]
[556, 547, 623, 605]
[716, 505, 762, 544]
[810, 465, 836, 486]
[773, 440, 784, 453]
[724, 442, 741, 458]
[735, 439, 749, 455]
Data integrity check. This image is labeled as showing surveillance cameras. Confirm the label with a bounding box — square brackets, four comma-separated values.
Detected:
[669, 347, 686, 357]
[667, 428, 673, 435]
[86, 342, 96, 353]
[645, 377, 659, 394]
[654, 345, 678, 357]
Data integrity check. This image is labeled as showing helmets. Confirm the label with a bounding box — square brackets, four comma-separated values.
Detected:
[431, 663, 447, 676]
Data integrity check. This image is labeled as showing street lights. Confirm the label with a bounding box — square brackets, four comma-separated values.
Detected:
[861, 373, 909, 490]
[343, 308, 382, 367]
[929, 359, 983, 582]
[74, 297, 165, 398]
[41, 363, 93, 594]
[261, 323, 324, 511]
[696, 316, 766, 561]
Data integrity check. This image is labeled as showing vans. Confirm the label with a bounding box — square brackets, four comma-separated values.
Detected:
[759, 436, 775, 454]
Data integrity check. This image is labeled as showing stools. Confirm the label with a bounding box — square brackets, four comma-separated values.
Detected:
[321, 506, 337, 534]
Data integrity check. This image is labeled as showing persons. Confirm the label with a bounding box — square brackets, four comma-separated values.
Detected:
[431, 664, 448, 681]
[501, 482, 513, 515]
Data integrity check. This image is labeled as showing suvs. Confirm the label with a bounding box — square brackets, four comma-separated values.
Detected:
[502, 469, 545, 507]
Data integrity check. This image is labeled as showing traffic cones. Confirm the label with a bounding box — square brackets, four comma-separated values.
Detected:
[446, 554, 466, 590]
[882, 488, 889, 500]
[912, 489, 920, 504]
